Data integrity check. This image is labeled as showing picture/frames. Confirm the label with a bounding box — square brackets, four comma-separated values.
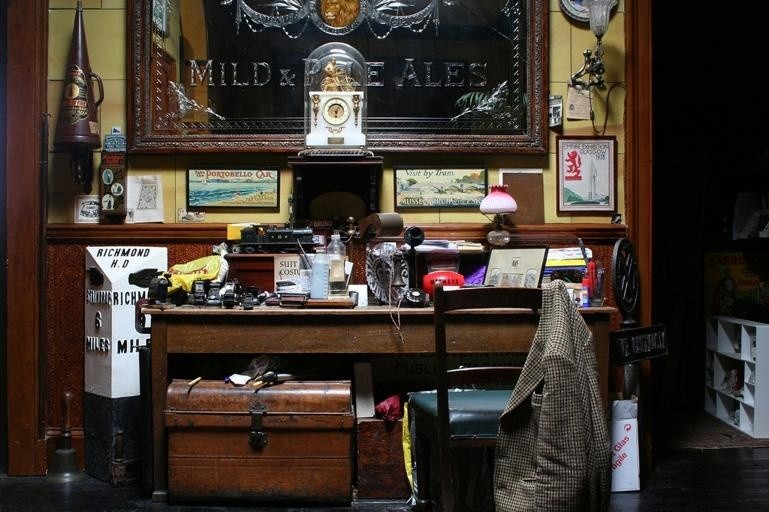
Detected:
[481, 243, 550, 288]
[392, 165, 488, 210]
[185, 166, 281, 210]
[122, 1, 550, 159]
[555, 135, 619, 214]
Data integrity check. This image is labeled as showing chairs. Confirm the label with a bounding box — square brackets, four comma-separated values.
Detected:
[406, 278, 543, 511]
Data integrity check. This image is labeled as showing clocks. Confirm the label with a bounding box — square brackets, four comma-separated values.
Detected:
[298, 42, 373, 157]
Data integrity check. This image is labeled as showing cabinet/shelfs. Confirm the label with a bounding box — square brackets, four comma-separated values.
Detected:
[703, 315, 768, 438]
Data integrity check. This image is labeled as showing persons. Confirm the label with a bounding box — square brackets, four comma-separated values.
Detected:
[322, 57, 341, 91]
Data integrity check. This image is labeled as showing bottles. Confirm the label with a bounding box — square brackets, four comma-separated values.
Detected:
[573, 287, 582, 308]
[310, 234, 346, 299]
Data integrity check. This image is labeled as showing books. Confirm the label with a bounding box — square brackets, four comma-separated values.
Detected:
[307, 295, 355, 308]
[541, 247, 593, 290]
[267, 296, 305, 307]
[457, 242, 482, 251]
[279, 290, 311, 300]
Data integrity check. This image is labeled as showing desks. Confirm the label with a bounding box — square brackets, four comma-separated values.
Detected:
[142, 302, 620, 505]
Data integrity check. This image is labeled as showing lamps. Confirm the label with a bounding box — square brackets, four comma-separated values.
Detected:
[568, 0, 619, 90]
[477, 184, 518, 245]
